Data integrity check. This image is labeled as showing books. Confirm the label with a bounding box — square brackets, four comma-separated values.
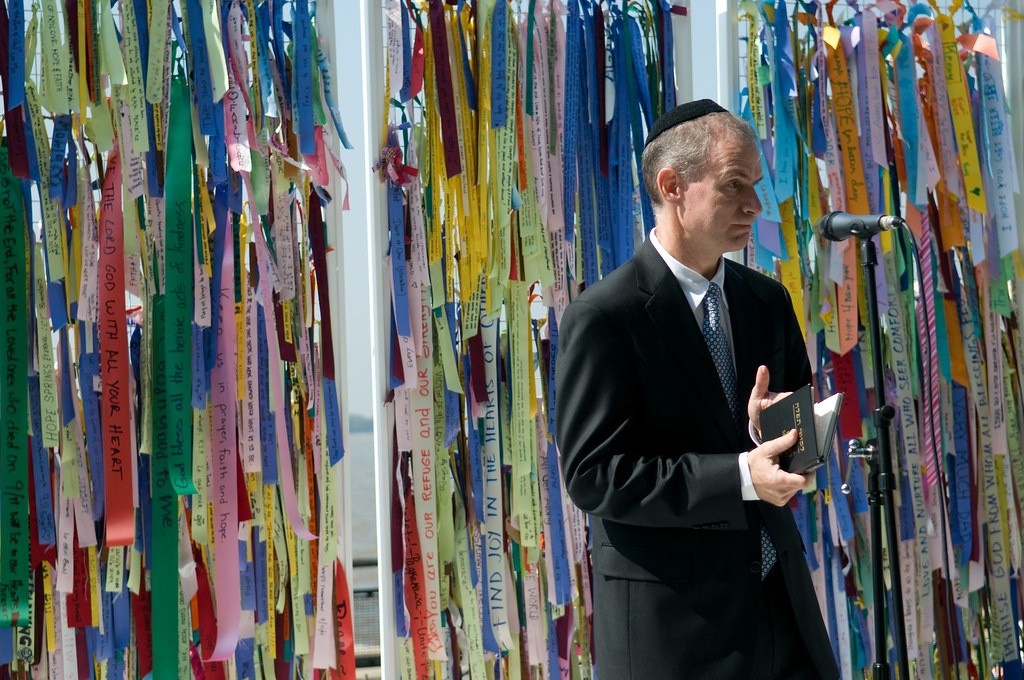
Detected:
[758, 384, 845, 475]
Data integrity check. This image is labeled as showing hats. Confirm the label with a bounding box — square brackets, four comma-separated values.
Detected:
[645, 99, 729, 147]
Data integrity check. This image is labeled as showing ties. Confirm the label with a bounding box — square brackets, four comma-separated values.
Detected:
[701, 283, 776, 581]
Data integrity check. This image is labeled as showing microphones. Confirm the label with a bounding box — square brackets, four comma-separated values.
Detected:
[820, 210, 905, 241]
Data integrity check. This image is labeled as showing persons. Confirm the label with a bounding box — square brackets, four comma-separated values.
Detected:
[555, 100, 841, 680]
